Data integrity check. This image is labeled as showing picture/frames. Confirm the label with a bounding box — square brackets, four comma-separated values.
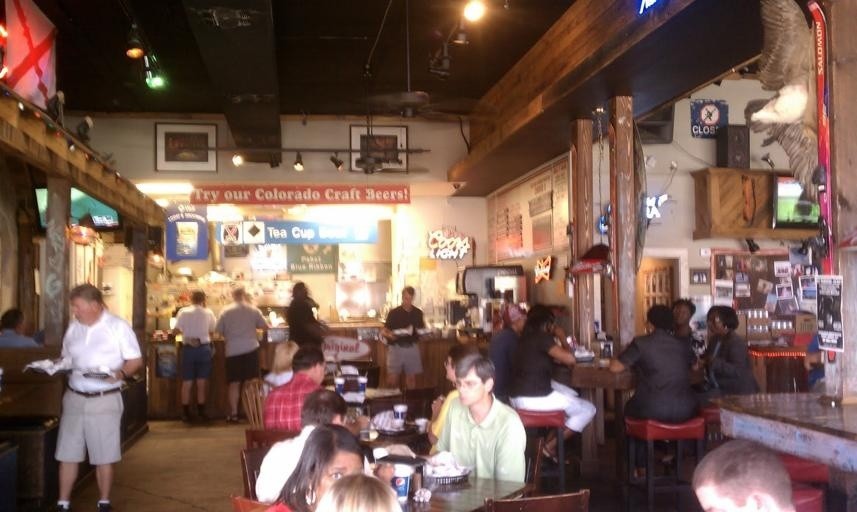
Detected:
[155, 122, 218, 172]
[349, 123, 409, 173]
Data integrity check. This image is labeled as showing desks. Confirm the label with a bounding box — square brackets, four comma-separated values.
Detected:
[570, 358, 710, 478]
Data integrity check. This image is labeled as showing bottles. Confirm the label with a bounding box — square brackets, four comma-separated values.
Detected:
[746, 320, 793, 333]
[737, 309, 769, 320]
[603, 343, 611, 358]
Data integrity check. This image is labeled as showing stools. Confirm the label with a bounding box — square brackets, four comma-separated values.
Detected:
[775, 451, 829, 483]
[624, 417, 705, 512]
[792, 485, 824, 511]
[698, 404, 722, 423]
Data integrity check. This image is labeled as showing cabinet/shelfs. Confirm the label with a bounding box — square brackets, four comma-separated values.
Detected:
[689, 167, 820, 240]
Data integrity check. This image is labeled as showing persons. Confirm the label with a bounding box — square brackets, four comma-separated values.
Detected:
[215, 287, 271, 424]
[610, 304, 697, 477]
[54, 283, 144, 511]
[316, 475, 404, 512]
[256, 389, 394, 503]
[0, 307, 42, 349]
[383, 286, 426, 389]
[426, 345, 527, 483]
[701, 306, 759, 399]
[691, 439, 796, 512]
[489, 303, 597, 464]
[171, 291, 218, 423]
[671, 298, 706, 357]
[262, 341, 369, 438]
[288, 283, 325, 347]
[269, 424, 364, 512]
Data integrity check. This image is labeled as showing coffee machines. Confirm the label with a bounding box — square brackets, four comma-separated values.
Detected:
[463, 263, 528, 338]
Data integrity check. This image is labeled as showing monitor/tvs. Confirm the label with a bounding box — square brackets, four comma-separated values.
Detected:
[771, 170, 819, 228]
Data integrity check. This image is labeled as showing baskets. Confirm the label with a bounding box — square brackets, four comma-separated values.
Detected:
[428, 474, 468, 486]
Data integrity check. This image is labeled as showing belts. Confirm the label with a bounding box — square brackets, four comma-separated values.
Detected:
[68, 386, 121, 397]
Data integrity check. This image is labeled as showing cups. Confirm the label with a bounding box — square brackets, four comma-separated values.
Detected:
[391, 463, 414, 502]
[257, 330, 263, 342]
[415, 418, 428, 434]
[333, 377, 345, 396]
[156, 333, 162, 340]
[356, 376, 368, 393]
[168, 332, 171, 340]
[392, 403, 407, 425]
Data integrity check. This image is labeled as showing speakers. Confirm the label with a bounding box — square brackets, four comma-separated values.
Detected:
[717, 124, 750, 168]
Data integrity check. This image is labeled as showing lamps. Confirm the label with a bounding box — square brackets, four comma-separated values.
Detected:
[231, 151, 378, 174]
[428, 0, 487, 77]
[121, 24, 164, 90]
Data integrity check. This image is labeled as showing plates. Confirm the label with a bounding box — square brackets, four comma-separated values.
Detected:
[31, 367, 72, 377]
[425, 475, 469, 486]
[575, 356, 595, 362]
[83, 372, 109, 380]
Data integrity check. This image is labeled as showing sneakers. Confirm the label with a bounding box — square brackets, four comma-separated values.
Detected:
[543, 445, 569, 465]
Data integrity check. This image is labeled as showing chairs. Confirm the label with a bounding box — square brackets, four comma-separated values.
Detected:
[228, 378, 301, 512]
[364, 384, 592, 512]
